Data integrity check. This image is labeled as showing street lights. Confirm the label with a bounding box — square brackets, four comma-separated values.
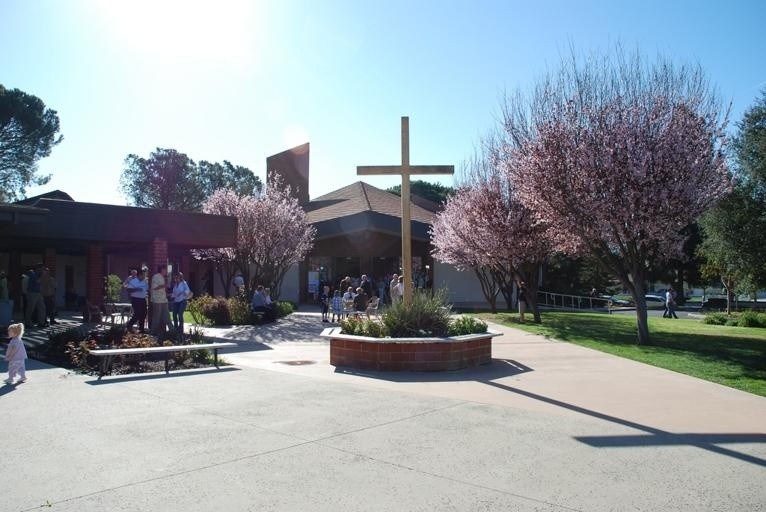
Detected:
[184, 292, 193, 299]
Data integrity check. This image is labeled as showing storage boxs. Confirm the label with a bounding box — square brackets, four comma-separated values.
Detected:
[253, 311, 267, 326]
[89, 343, 238, 379]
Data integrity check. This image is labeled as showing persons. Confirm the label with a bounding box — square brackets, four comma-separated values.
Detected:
[665, 287, 674, 318]
[663, 288, 680, 318]
[518, 280, 530, 323]
[2, 320, 27, 386]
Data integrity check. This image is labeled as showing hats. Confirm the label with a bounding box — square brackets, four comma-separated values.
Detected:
[599, 294, 631, 307]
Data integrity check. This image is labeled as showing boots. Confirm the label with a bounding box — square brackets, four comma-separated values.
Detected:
[643, 293, 678, 309]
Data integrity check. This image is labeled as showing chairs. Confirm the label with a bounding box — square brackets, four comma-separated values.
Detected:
[17, 378, 27, 382]
[4, 379, 14, 382]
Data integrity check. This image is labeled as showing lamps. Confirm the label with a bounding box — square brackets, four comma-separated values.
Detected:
[85, 299, 147, 330]
[328, 296, 380, 321]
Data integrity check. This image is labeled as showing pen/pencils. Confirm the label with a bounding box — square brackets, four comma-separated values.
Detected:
[698, 297, 727, 309]
